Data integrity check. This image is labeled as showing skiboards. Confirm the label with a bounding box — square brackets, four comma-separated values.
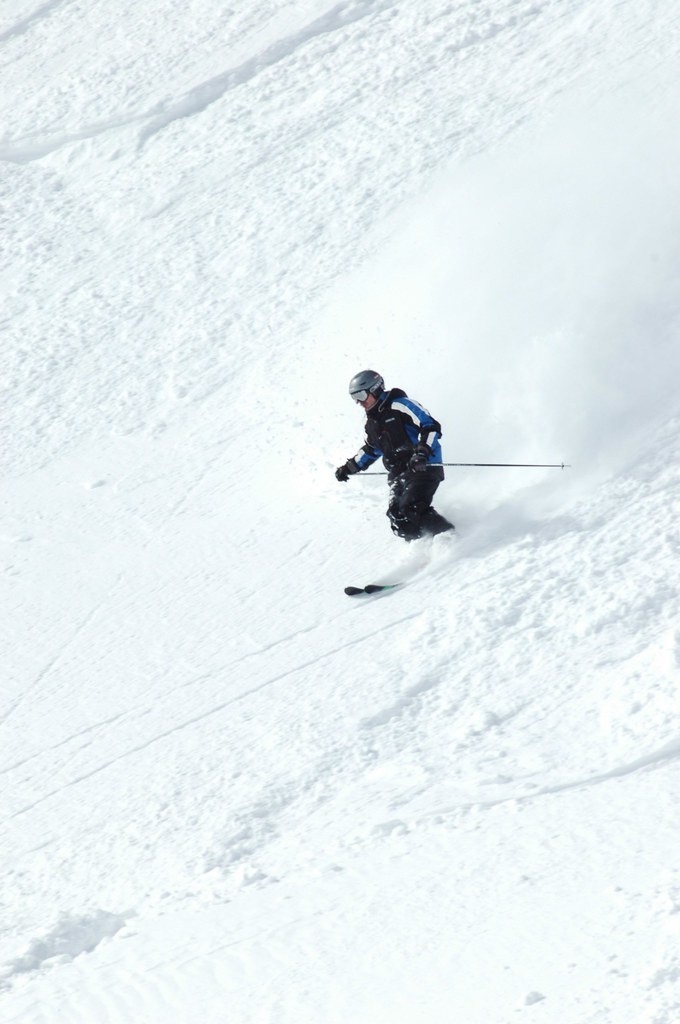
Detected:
[343, 581, 403, 596]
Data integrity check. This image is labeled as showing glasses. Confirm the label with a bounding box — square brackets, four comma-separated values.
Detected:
[350, 389, 369, 404]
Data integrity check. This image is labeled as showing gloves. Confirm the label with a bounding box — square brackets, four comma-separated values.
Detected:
[334, 464, 352, 482]
[409, 450, 427, 474]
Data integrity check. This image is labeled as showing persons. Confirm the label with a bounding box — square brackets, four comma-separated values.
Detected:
[335, 370, 455, 543]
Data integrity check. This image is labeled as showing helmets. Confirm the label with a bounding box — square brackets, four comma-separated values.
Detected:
[349, 370, 381, 394]
